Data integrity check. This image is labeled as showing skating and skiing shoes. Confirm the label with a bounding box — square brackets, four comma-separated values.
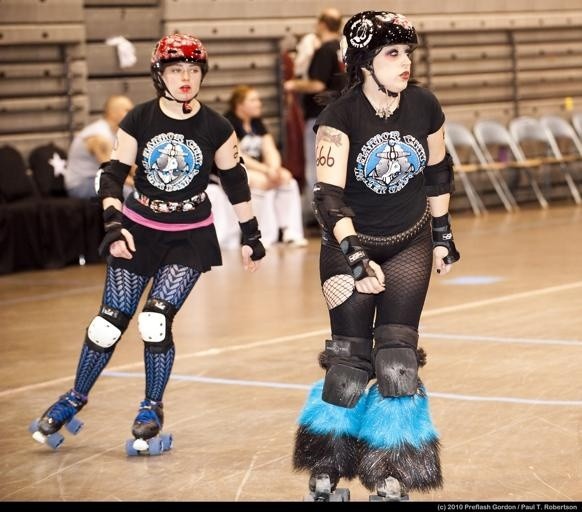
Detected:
[125, 398, 172, 457]
[369, 476, 408, 502]
[28, 388, 87, 448]
[303, 465, 349, 502]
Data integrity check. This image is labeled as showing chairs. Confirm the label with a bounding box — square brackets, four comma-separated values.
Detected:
[444, 109, 582, 222]
[29, 139, 112, 267]
[1, 140, 84, 274]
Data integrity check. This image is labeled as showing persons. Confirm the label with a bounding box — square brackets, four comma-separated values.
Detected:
[39, 33, 265, 438]
[223, 86, 310, 251]
[65, 96, 138, 200]
[293, 11, 460, 496]
[283, 9, 346, 197]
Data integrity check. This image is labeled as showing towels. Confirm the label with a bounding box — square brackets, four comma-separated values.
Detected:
[105, 34, 137, 69]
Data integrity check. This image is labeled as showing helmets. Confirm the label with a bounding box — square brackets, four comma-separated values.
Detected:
[151, 33, 208, 71]
[340, 10, 418, 68]
